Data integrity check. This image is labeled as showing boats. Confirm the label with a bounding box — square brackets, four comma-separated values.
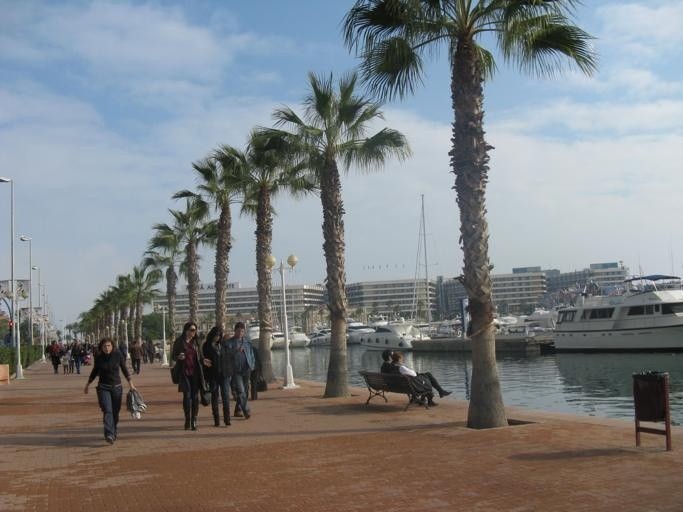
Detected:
[552, 257, 682, 356]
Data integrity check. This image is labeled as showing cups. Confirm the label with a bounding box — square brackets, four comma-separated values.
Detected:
[180, 353, 184, 359]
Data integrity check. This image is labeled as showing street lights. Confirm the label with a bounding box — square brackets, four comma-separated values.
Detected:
[33, 262, 55, 365]
[154, 304, 168, 366]
[17, 232, 36, 348]
[0, 176, 29, 382]
[59, 319, 66, 340]
[85, 319, 128, 348]
[260, 252, 298, 392]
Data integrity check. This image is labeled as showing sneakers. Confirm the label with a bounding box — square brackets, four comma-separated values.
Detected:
[183, 412, 251, 431]
[439, 390, 452, 398]
[428, 402, 439, 407]
[106, 431, 118, 445]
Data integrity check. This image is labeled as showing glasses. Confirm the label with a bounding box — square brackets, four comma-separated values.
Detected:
[187, 329, 196, 332]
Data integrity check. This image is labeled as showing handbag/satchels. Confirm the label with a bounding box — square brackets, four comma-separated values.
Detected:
[170, 359, 184, 385]
[200, 391, 212, 408]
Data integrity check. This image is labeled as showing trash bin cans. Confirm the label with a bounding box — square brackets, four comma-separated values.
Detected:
[631, 371, 671, 451]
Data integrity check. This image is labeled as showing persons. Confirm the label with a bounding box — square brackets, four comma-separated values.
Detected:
[381, 349, 422, 404]
[392, 352, 452, 407]
[45, 324, 261, 443]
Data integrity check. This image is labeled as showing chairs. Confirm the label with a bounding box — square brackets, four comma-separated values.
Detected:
[358, 368, 432, 412]
